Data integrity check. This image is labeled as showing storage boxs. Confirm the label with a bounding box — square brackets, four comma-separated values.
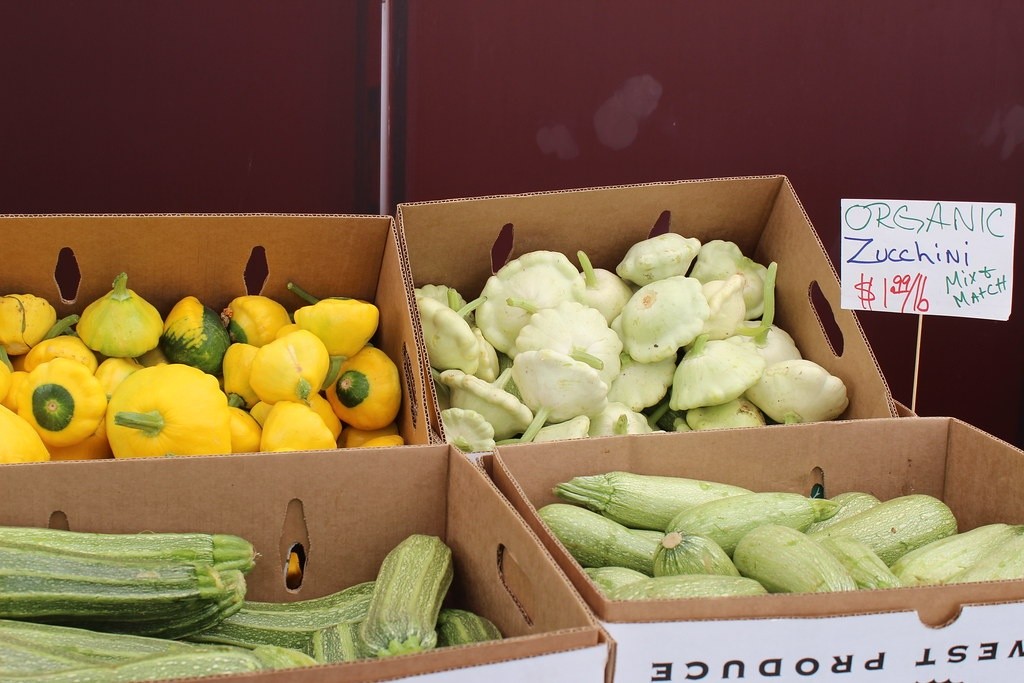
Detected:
[0, 175, 1024, 683]
